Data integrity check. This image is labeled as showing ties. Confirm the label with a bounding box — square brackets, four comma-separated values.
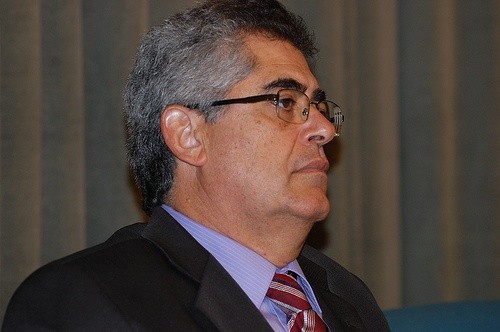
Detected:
[266, 272, 329, 332]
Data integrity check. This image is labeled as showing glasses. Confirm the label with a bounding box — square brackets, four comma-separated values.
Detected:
[179, 88, 345, 133]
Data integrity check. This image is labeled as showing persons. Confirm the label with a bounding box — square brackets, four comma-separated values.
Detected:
[0, 0, 391, 332]
